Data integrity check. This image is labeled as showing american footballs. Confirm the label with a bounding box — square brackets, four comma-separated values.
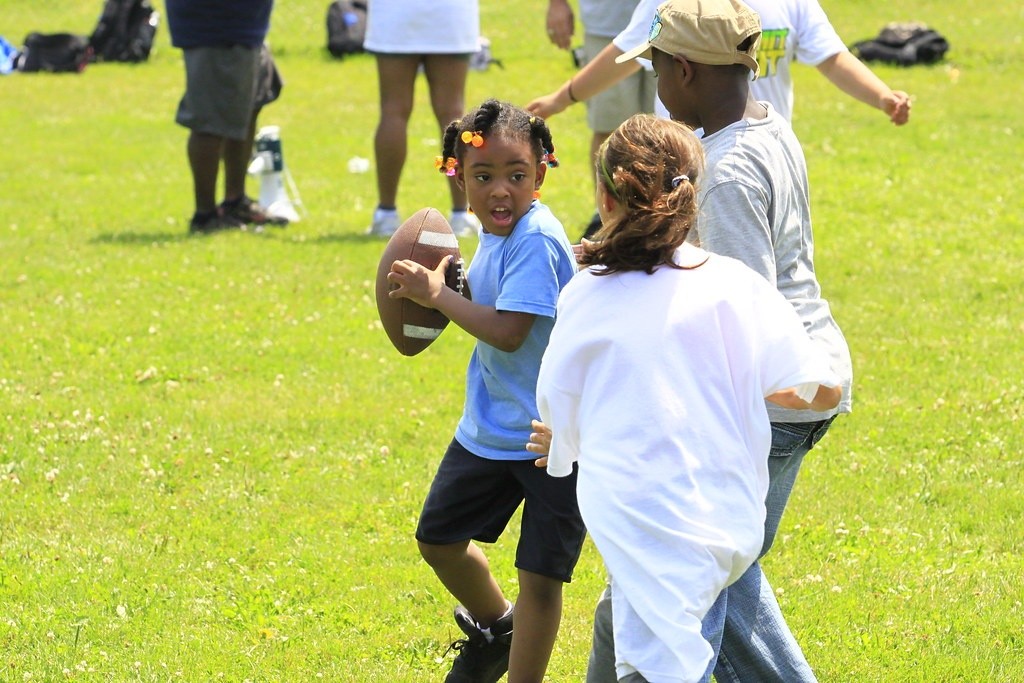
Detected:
[377, 208, 464, 356]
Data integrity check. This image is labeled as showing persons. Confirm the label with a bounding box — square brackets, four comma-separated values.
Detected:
[359, 0, 487, 243]
[542, 0, 661, 214]
[385, 94, 594, 683]
[162, 0, 288, 234]
[521, 1, 914, 161]
[614, 2, 858, 683]
[523, 114, 844, 683]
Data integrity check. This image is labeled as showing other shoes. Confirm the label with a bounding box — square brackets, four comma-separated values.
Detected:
[450, 212, 482, 236]
[217, 197, 289, 227]
[189, 207, 222, 234]
[365, 207, 400, 237]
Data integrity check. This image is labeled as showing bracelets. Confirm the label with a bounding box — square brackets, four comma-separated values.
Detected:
[564, 78, 581, 103]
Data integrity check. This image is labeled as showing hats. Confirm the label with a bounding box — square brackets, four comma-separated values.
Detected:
[614, 0, 763, 82]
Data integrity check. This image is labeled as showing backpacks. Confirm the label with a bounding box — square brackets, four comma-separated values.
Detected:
[89, 0, 158, 63]
[325, 0, 367, 57]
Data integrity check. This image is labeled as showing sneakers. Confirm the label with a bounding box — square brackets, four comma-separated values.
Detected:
[442, 599, 515, 682]
